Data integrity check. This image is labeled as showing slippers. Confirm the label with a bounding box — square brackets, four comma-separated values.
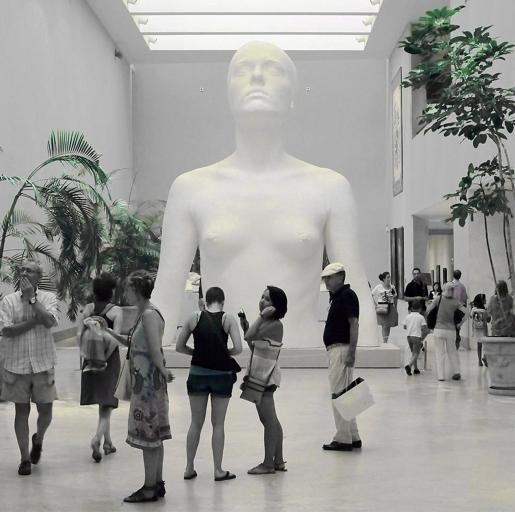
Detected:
[215, 471, 237, 481]
[184, 471, 197, 480]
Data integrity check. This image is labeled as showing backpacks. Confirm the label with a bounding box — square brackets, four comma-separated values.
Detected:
[78, 315, 120, 374]
[472, 310, 488, 329]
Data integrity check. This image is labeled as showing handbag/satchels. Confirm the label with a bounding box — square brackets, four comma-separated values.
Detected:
[376, 302, 389, 315]
[114, 360, 135, 402]
[240, 377, 266, 406]
[331, 375, 376, 422]
[426, 305, 439, 330]
[228, 356, 242, 374]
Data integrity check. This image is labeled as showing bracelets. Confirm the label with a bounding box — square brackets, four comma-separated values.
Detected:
[260, 312, 265, 320]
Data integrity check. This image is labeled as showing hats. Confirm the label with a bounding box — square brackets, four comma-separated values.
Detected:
[318, 262, 345, 277]
[16, 262, 42, 280]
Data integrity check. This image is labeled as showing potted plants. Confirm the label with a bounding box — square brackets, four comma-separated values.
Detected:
[396, 7, 515, 396]
[103, 194, 166, 332]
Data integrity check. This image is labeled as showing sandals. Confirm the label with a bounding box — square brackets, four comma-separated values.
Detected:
[122, 485, 157, 502]
[248, 464, 275, 474]
[275, 460, 287, 471]
[156, 480, 166, 497]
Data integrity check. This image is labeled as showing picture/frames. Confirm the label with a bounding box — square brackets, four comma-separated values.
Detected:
[391, 66, 403, 196]
[389, 229, 395, 296]
[395, 226, 405, 300]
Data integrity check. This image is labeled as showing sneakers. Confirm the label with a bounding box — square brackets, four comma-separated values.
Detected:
[413, 368, 420, 374]
[30, 432, 42, 465]
[18, 460, 31, 476]
[405, 364, 412, 376]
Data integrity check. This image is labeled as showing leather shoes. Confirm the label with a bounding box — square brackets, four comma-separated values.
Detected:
[321, 442, 351, 451]
[350, 440, 363, 448]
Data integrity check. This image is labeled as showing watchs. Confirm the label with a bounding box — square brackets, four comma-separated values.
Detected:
[29, 296, 37, 304]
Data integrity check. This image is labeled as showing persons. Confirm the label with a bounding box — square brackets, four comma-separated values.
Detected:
[488, 280, 512, 335]
[372, 272, 398, 343]
[321, 262, 361, 451]
[403, 300, 428, 375]
[0, 262, 59, 475]
[403, 267, 429, 351]
[176, 286, 242, 481]
[427, 282, 442, 333]
[152, 40, 378, 351]
[77, 272, 121, 461]
[427, 282, 469, 381]
[450, 270, 467, 348]
[100, 270, 175, 502]
[469, 294, 491, 366]
[239, 286, 287, 474]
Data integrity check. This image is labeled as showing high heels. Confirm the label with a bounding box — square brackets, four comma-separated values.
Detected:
[103, 441, 116, 455]
[90, 437, 102, 463]
